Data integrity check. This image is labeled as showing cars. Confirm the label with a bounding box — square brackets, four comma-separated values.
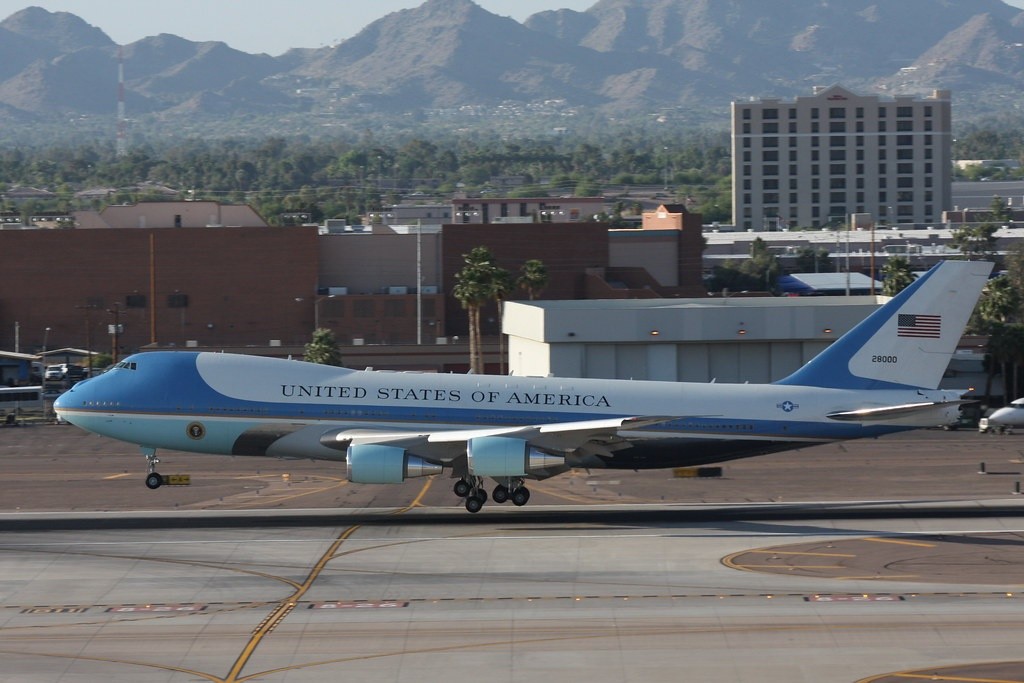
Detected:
[61, 365, 104, 383]
[45, 365, 64, 380]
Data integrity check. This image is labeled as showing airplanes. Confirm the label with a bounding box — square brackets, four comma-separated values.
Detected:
[976, 395, 1024, 433]
[50, 260, 997, 516]
